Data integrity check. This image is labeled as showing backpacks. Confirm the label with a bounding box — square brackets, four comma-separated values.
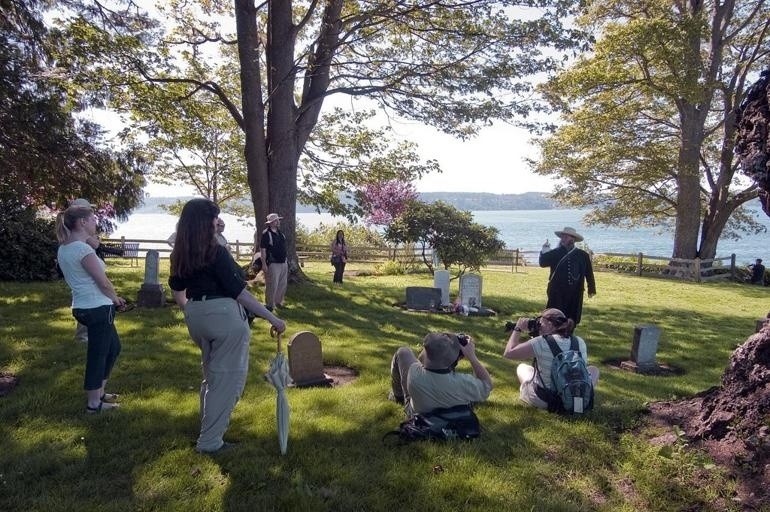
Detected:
[533, 334, 594, 414]
[382, 404, 480, 448]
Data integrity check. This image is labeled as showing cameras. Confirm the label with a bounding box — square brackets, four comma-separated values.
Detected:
[96, 243, 126, 259]
[504, 316, 540, 336]
[457, 335, 468, 356]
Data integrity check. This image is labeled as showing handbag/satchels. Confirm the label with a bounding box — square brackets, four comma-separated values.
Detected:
[331, 255, 343, 266]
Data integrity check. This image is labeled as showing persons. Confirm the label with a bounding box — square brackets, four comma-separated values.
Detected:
[260, 212, 289, 313]
[330, 230, 350, 284]
[167, 222, 178, 251]
[539, 226, 597, 335]
[168, 198, 287, 452]
[56, 204, 122, 416]
[744, 258, 766, 285]
[502, 309, 600, 411]
[215, 218, 233, 256]
[73, 198, 100, 343]
[388, 330, 494, 418]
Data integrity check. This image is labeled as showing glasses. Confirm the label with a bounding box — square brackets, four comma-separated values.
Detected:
[115, 301, 136, 314]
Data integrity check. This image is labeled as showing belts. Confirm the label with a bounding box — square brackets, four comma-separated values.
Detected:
[192, 295, 230, 301]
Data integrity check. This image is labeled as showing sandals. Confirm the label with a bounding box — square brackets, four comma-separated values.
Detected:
[85, 402, 117, 414]
[100, 393, 117, 402]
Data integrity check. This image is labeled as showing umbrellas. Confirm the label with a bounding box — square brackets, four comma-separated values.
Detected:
[264, 325, 294, 456]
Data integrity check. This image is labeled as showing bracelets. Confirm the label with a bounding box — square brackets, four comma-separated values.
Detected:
[514, 326, 523, 333]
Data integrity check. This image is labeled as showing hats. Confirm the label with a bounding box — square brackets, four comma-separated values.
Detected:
[419, 331, 460, 369]
[555, 227, 584, 242]
[70, 199, 97, 208]
[265, 213, 284, 224]
